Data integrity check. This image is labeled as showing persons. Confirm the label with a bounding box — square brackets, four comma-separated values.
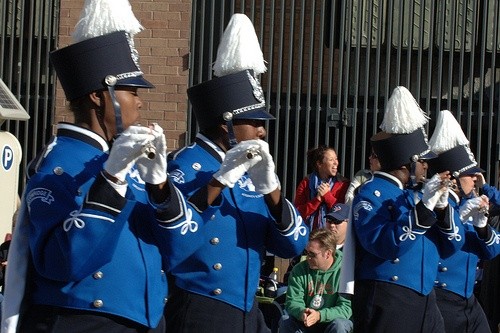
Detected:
[278, 228, 353, 333]
[29, 0, 206, 333]
[324, 203, 351, 251]
[165, 13, 308, 333]
[425, 111, 500, 333]
[294, 147, 349, 232]
[344, 147, 382, 205]
[352, 86, 465, 333]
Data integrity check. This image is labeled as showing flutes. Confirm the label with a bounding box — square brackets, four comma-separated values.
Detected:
[245, 150, 261, 159]
[420, 176, 458, 188]
[136, 121, 156, 161]
[472, 185, 489, 217]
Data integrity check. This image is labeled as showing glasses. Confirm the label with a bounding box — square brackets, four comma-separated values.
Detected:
[371, 151, 378, 159]
[302, 248, 327, 258]
[326, 217, 343, 224]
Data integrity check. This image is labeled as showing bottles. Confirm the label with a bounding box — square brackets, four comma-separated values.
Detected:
[269, 267, 280, 283]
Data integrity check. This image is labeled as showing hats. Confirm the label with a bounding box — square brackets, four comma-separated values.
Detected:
[371, 86, 439, 171]
[187, 13, 276, 128]
[49, 0, 156, 100]
[323, 204, 350, 221]
[428, 110, 486, 180]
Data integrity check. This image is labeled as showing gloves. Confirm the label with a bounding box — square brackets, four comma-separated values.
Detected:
[104, 125, 160, 182]
[213, 140, 262, 188]
[249, 139, 279, 195]
